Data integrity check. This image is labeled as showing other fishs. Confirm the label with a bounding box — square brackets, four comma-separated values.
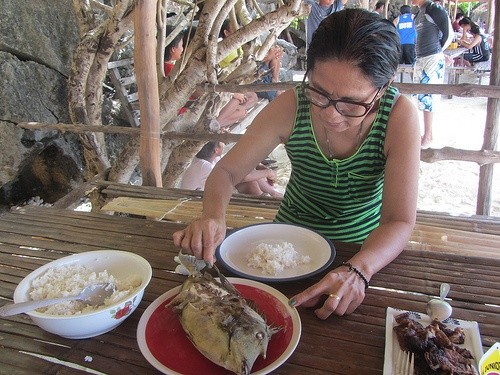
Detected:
[174, 248, 283, 375]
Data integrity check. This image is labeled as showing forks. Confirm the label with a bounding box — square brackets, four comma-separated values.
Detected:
[213, 263, 241, 295]
[395, 350, 415, 375]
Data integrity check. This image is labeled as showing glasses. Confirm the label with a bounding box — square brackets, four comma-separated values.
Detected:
[298, 58, 387, 117]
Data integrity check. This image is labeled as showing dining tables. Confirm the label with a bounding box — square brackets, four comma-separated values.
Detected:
[441, 48, 468, 78]
[0, 204, 500, 375]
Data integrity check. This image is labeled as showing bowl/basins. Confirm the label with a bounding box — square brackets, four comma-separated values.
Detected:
[12, 248, 153, 340]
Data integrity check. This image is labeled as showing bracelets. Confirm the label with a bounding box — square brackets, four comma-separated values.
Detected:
[341, 262, 369, 289]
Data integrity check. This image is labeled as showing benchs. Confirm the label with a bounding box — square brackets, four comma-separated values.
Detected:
[88, 179, 500, 259]
[394, 65, 464, 84]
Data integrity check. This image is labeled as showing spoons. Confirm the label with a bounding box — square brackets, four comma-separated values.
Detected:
[0, 281, 117, 320]
[425, 282, 452, 322]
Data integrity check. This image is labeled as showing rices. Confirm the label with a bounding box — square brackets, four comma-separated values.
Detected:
[29, 263, 143, 316]
[247, 242, 311, 277]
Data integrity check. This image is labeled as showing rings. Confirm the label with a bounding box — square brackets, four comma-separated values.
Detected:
[329, 294, 341, 301]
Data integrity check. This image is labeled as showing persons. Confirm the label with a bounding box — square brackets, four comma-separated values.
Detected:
[177, 7, 423, 320]
[460, 25, 491, 67]
[390, 5, 417, 80]
[303, 0, 348, 51]
[183, 26, 247, 125]
[374, 2, 385, 17]
[220, 15, 285, 101]
[160, 25, 195, 113]
[180, 136, 284, 198]
[452, 12, 464, 32]
[409, 0, 454, 149]
[460, 17, 485, 41]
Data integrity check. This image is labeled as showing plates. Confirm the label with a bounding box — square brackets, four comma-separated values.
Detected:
[136, 277, 302, 375]
[217, 221, 336, 282]
[383, 307, 484, 375]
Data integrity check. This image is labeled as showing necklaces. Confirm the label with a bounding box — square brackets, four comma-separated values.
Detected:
[323, 119, 363, 160]
